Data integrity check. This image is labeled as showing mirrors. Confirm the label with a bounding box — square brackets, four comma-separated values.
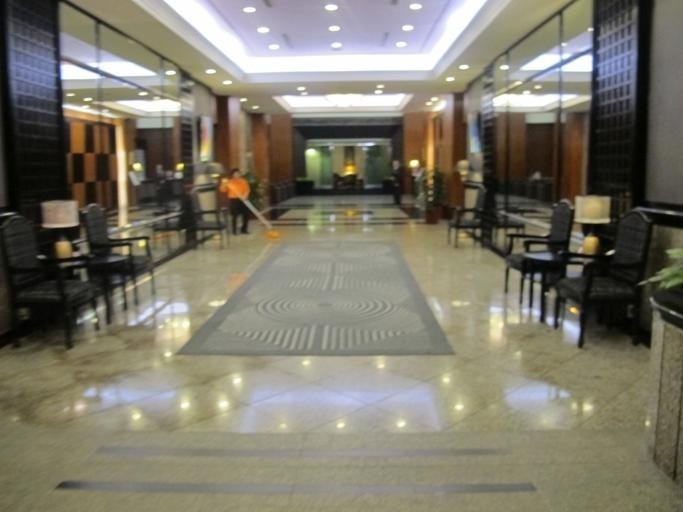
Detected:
[493, 0, 595, 241]
[58, 0, 187, 245]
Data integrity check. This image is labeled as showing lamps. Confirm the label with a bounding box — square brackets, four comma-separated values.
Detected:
[39, 198, 82, 261]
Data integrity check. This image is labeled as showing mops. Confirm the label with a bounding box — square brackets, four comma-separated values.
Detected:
[223, 179, 282, 241]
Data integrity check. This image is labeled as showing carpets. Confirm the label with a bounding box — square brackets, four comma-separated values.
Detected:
[175, 237, 457, 356]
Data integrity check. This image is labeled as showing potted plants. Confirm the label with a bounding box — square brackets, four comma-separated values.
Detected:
[417, 165, 445, 224]
[632, 244, 682, 486]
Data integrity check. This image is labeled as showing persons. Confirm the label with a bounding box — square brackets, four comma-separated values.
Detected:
[344, 158, 356, 178]
[220, 168, 251, 235]
[390, 160, 402, 205]
[155, 164, 166, 208]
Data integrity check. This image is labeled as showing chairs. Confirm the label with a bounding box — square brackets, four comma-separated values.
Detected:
[152, 179, 186, 249]
[489, 186, 526, 251]
[448, 187, 489, 249]
[186, 189, 231, 249]
[0, 203, 158, 351]
[502, 198, 655, 349]
